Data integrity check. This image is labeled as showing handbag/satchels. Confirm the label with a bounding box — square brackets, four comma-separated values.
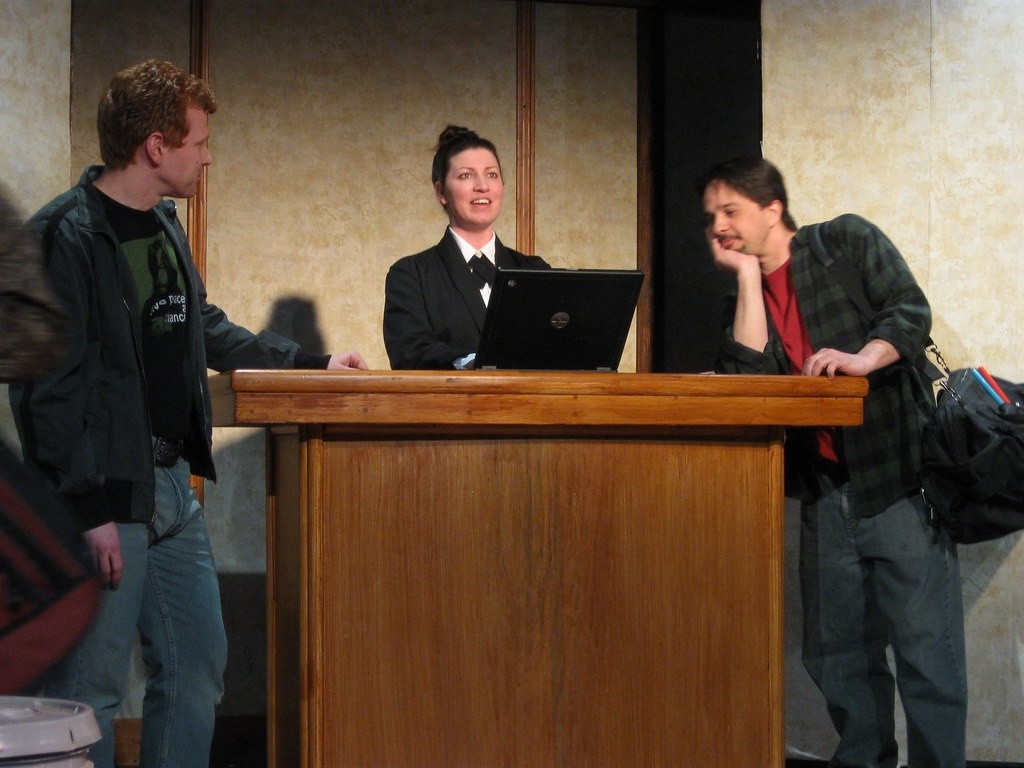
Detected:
[919, 368, 1024, 544]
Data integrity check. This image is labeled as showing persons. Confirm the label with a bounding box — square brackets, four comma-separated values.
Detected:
[383, 126, 551, 373]
[0, 58, 368, 768]
[701, 159, 968, 768]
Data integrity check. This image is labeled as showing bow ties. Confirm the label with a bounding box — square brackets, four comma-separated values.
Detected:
[468, 254, 497, 289]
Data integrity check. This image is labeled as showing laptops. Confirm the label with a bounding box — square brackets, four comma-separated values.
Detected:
[473, 266, 645, 371]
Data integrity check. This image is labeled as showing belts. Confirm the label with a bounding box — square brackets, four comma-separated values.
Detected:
[152, 437, 185, 467]
